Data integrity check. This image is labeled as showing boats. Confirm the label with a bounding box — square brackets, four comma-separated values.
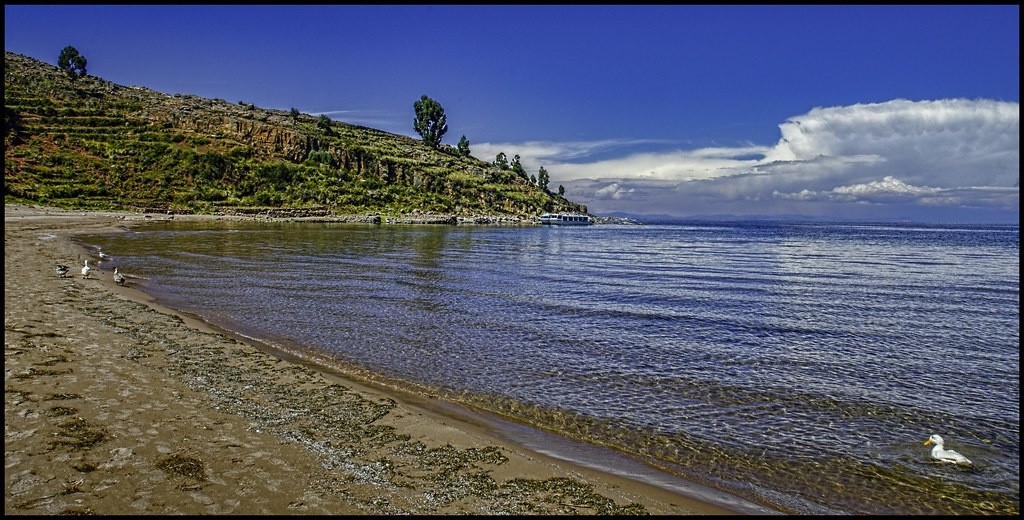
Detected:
[540, 213, 595, 225]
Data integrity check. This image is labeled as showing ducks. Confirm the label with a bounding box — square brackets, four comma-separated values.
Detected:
[924, 434, 975, 469]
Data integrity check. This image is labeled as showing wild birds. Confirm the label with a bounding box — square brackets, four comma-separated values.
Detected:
[55, 264, 70, 277]
[113, 266, 126, 284]
[81, 260, 92, 276]
